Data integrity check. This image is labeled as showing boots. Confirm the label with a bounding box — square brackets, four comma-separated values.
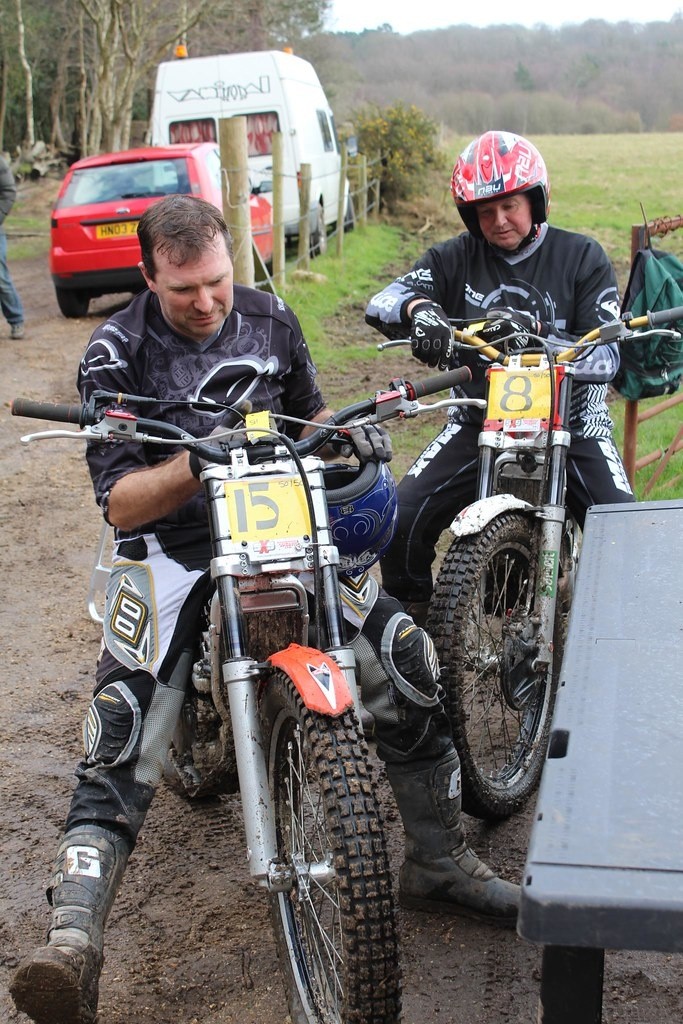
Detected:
[386, 747, 522, 929]
[10, 824, 131, 1024]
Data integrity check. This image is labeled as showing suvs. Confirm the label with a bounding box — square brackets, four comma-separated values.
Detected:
[50, 142, 274, 321]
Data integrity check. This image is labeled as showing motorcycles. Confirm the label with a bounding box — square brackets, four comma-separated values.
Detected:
[376, 307, 683, 818]
[11, 362, 488, 1024]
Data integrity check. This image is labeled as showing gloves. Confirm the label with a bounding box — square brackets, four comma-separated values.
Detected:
[325, 413, 393, 462]
[189, 400, 294, 482]
[410, 301, 455, 371]
[475, 306, 538, 355]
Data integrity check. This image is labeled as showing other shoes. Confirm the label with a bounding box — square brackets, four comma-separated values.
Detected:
[12, 321, 24, 339]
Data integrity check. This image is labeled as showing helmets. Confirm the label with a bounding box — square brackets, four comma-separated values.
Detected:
[450, 131, 551, 239]
[321, 461, 397, 577]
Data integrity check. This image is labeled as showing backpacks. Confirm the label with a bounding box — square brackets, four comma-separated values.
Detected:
[609, 224, 683, 401]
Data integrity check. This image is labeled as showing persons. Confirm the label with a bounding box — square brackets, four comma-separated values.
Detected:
[9, 192, 523, 1024]
[0, 155, 26, 339]
[363, 129, 625, 735]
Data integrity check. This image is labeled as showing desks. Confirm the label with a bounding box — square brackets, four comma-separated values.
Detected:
[515, 498, 683, 1023]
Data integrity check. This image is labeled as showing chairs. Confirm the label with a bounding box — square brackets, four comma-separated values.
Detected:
[98, 173, 135, 202]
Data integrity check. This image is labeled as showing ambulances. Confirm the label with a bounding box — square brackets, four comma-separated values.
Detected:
[149, 46, 356, 257]
